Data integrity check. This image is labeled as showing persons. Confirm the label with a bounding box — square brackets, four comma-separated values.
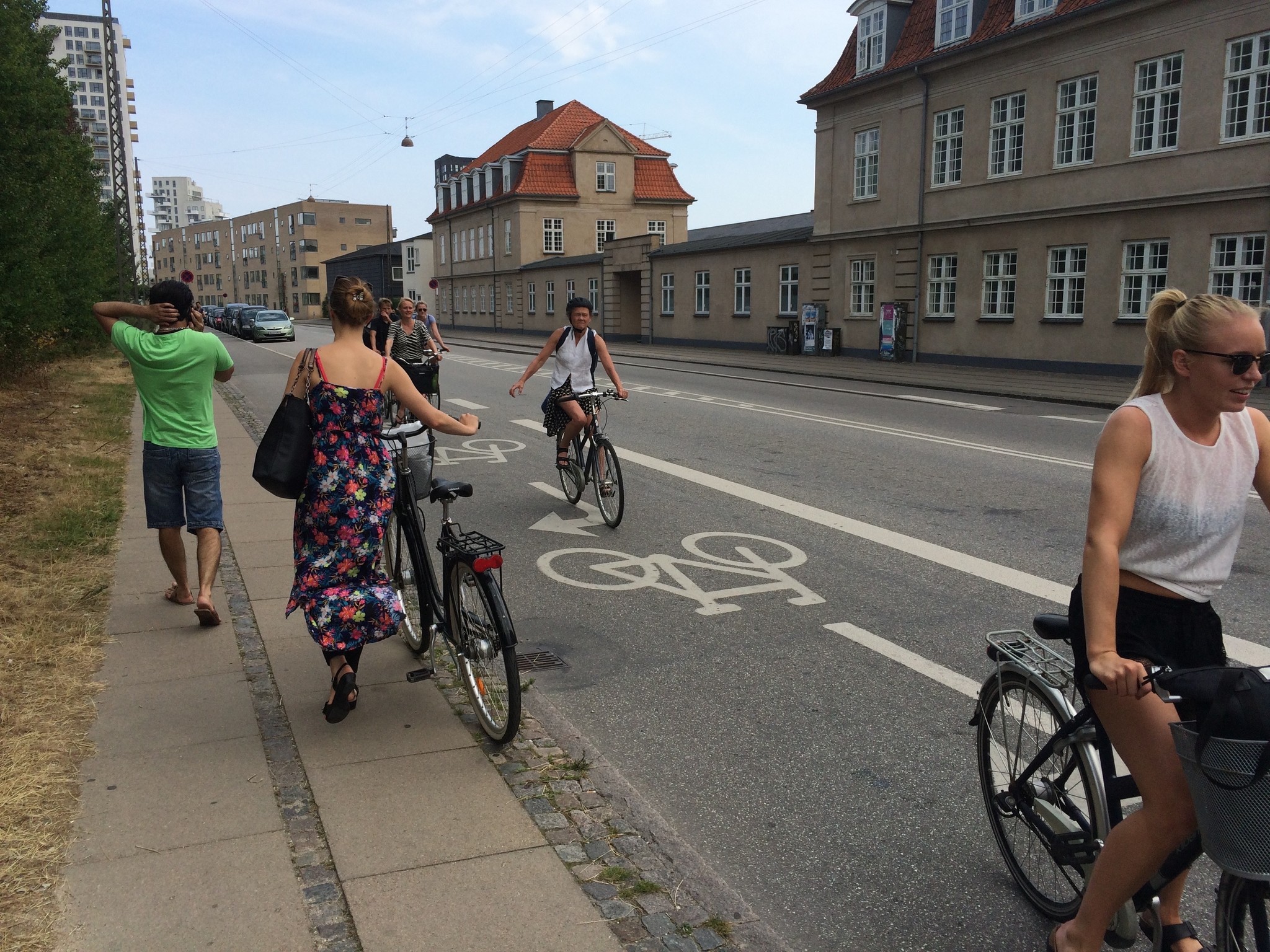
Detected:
[371, 297, 450, 425]
[1049, 288, 1270, 952]
[509, 297, 629, 496]
[286, 277, 478, 724]
[93, 280, 235, 626]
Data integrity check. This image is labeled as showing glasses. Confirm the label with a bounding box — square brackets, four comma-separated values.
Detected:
[331, 275, 374, 295]
[1176, 348, 1270, 375]
[418, 308, 427, 312]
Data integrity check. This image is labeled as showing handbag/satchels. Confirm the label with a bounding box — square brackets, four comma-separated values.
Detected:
[251, 345, 319, 501]
[1140, 664, 1270, 792]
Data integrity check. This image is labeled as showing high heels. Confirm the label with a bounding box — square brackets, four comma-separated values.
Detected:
[321, 662, 357, 724]
[348, 684, 359, 711]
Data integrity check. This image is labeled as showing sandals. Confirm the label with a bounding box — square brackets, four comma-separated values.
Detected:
[598, 480, 612, 496]
[555, 444, 569, 469]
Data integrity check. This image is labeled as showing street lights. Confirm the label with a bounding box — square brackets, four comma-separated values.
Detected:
[178, 268, 190, 282]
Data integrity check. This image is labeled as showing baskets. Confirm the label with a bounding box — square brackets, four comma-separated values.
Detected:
[384, 433, 436, 502]
[1166, 719, 1269, 883]
[400, 364, 440, 395]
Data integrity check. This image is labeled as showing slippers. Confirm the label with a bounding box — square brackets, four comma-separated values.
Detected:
[193, 604, 220, 627]
[395, 408, 406, 425]
[164, 584, 196, 606]
[1046, 923, 1064, 952]
[1137, 911, 1213, 952]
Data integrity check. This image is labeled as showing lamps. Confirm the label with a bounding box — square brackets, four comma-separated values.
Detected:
[401, 117, 414, 147]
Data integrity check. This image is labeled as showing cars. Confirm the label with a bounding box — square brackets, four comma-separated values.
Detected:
[200, 302, 269, 340]
[249, 309, 295, 343]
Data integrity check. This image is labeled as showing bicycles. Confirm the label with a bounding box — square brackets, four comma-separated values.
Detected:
[554, 388, 629, 528]
[966, 610, 1270, 952]
[378, 347, 449, 428]
[378, 412, 522, 745]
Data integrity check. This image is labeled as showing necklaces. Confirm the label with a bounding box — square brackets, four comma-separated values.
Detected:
[156, 327, 187, 330]
